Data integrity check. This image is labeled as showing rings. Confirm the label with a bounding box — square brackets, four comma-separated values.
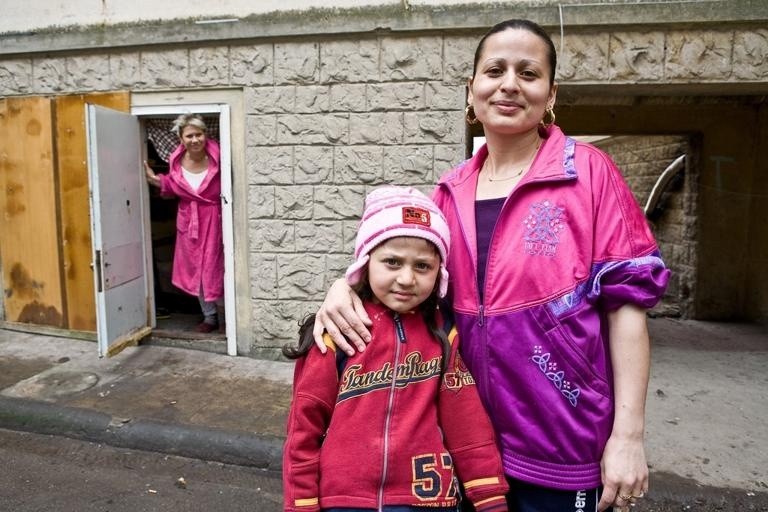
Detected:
[615, 492, 633, 501]
[629, 489, 645, 503]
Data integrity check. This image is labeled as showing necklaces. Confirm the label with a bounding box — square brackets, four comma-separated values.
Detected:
[483, 137, 542, 183]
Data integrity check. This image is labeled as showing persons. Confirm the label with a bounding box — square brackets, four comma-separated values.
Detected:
[141, 112, 221, 334]
[280, 186, 511, 511]
[312, 20, 672, 511]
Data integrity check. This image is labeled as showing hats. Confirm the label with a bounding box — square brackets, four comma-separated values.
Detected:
[345, 185, 451, 298]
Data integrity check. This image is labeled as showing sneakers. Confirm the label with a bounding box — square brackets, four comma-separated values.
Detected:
[218, 324, 225, 334]
[196, 321, 212, 332]
